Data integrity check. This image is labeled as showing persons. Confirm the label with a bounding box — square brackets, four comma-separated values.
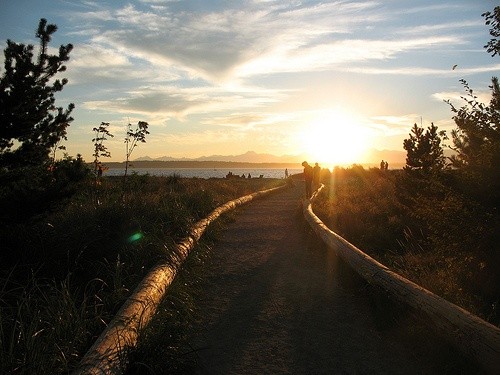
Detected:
[242, 174, 245, 178]
[313, 163, 320, 187]
[248, 172, 251, 179]
[380, 160, 385, 169]
[385, 162, 388, 169]
[289, 174, 291, 177]
[228, 172, 232, 176]
[302, 161, 313, 199]
[285, 169, 288, 178]
[258, 175, 263, 179]
